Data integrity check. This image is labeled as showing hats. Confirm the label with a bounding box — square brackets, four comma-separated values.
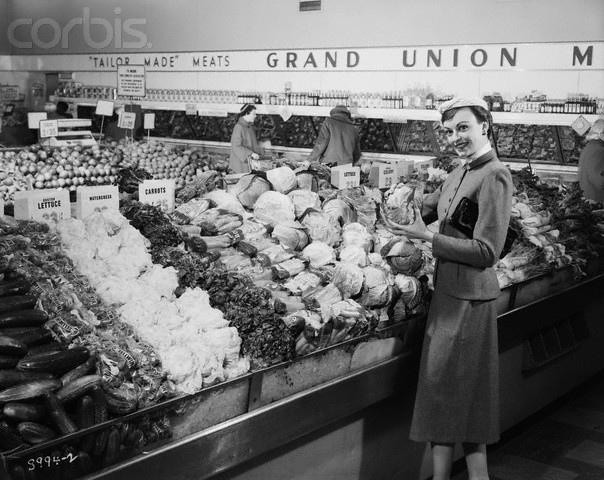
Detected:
[331, 105, 351, 115]
[437, 94, 489, 114]
[238, 102, 248, 112]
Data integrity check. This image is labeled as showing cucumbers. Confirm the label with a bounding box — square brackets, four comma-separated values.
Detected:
[0, 268, 136, 480]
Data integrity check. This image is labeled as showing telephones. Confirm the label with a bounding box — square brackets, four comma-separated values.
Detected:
[0, 104, 15, 116]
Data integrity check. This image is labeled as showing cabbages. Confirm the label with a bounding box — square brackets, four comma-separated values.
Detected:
[235, 166, 424, 328]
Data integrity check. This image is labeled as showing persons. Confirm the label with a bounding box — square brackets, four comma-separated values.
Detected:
[0, 109, 38, 147]
[307, 105, 362, 166]
[228, 104, 260, 174]
[578, 119, 604, 204]
[384, 97, 512, 480]
[104, 106, 136, 140]
[47, 101, 84, 140]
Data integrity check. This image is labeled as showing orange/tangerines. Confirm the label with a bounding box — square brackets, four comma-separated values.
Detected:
[116, 140, 198, 190]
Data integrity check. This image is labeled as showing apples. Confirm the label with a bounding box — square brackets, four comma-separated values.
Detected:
[17, 143, 118, 193]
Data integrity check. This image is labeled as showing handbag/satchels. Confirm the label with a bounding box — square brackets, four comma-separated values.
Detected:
[449, 196, 519, 260]
